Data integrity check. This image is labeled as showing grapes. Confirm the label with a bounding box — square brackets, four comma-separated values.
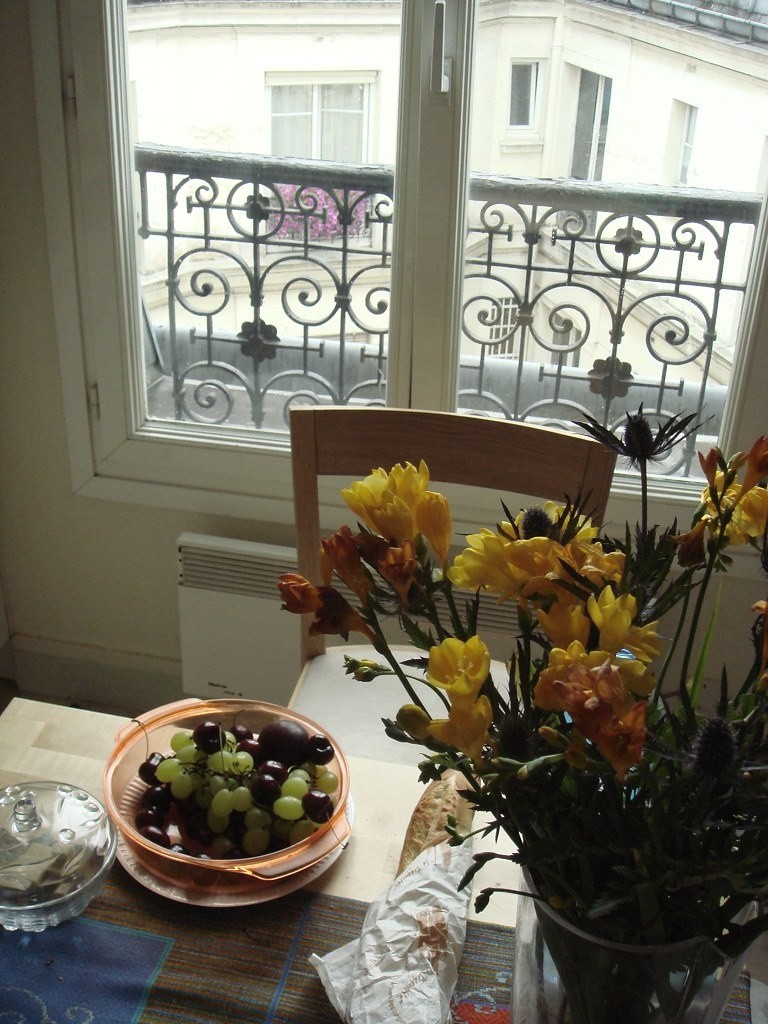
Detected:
[155, 731, 340, 856]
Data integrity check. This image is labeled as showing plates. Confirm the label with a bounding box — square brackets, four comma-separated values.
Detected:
[115, 798, 355, 908]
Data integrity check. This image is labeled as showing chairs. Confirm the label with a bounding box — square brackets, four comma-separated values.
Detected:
[282, 403, 620, 770]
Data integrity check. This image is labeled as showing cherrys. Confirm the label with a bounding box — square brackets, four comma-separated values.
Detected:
[130, 708, 348, 857]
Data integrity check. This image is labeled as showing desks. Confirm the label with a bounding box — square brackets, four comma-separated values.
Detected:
[0, 698, 768, 1024]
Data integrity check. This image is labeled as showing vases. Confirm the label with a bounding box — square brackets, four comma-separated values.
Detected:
[508, 862, 762, 1024]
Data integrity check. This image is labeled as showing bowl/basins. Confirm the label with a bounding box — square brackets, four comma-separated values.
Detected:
[103, 698, 352, 881]
[0, 780, 118, 933]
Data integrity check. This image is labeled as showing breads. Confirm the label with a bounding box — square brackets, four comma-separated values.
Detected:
[393, 769, 478, 877]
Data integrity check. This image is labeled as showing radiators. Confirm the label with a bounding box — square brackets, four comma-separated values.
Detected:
[178, 529, 547, 708]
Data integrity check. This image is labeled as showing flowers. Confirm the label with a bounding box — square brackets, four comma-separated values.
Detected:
[271, 401, 768, 1024]
[267, 186, 369, 243]
